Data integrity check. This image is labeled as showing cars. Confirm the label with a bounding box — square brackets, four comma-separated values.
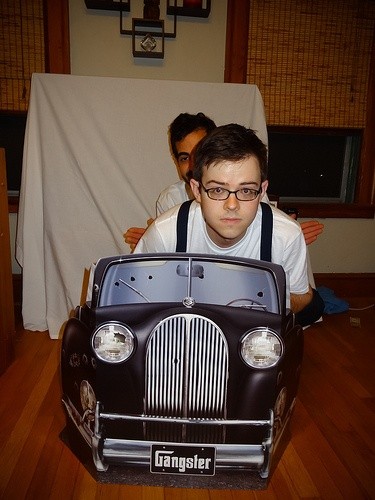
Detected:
[59, 252, 329, 481]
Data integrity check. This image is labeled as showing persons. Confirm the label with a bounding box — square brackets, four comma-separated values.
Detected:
[129, 123, 313, 314]
[124, 111, 325, 325]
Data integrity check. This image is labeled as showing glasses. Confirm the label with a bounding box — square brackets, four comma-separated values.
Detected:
[199, 181, 261, 201]
